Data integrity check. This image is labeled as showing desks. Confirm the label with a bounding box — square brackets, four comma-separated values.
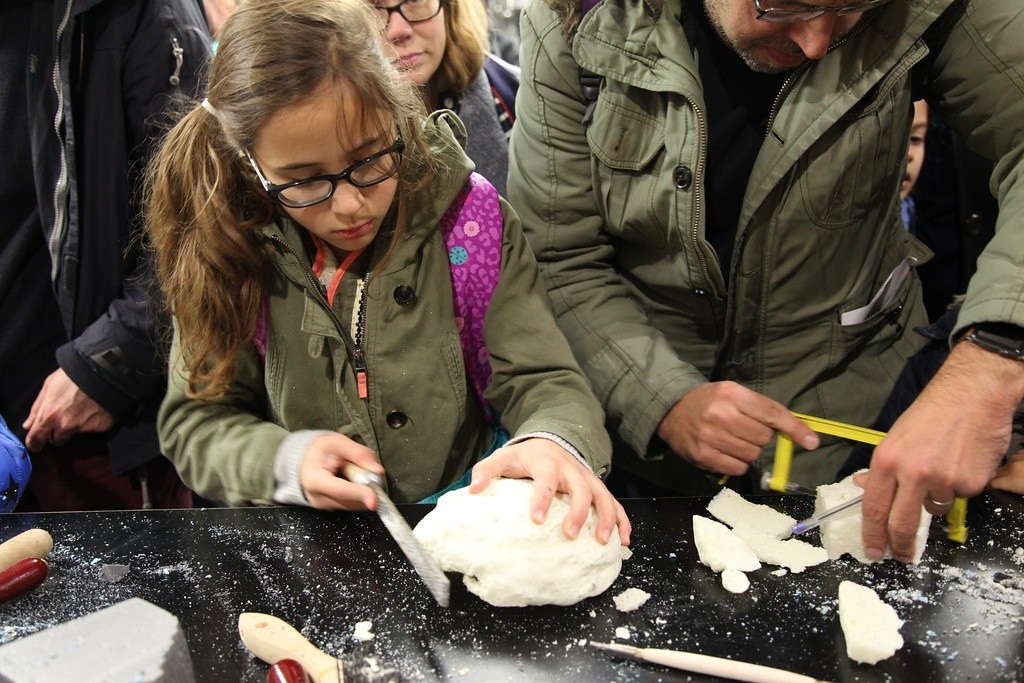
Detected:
[0, 497, 1024, 683]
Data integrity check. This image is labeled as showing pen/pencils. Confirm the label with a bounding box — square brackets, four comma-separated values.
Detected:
[792, 491, 865, 535]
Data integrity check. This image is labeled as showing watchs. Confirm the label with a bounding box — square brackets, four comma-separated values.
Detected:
[960, 322, 1023, 360]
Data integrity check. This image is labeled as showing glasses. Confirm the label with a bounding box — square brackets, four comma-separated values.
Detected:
[370, 0, 442, 30]
[754, 0, 887, 25]
[242, 124, 406, 208]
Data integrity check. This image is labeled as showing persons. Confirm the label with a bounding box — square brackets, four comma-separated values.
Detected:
[494, 0, 1024, 565]
[145, 2, 634, 559]
[901, 96, 928, 237]
[365, 2, 522, 198]
[1, 0, 212, 509]
